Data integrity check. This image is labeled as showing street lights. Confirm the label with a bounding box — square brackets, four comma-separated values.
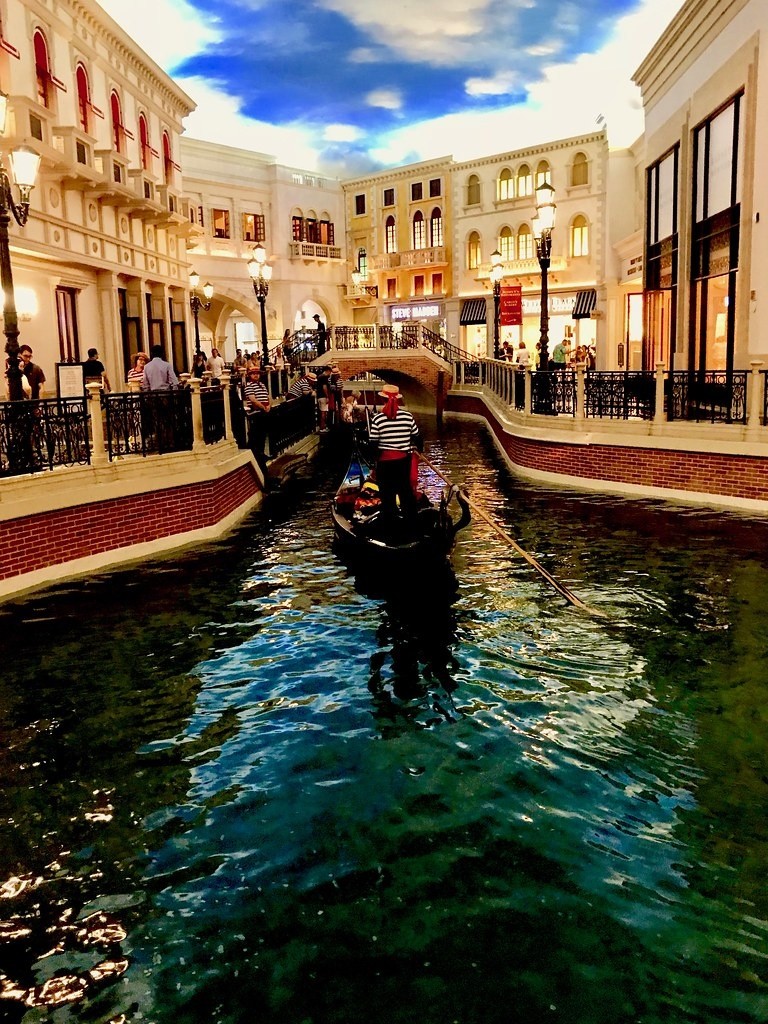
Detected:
[243, 240, 272, 383]
[531, 182, 558, 416]
[0, 145, 41, 399]
[351, 264, 365, 330]
[189, 270, 215, 381]
[484, 250, 505, 356]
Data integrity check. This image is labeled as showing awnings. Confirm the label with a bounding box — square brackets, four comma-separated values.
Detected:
[572, 290, 596, 319]
[460, 299, 486, 325]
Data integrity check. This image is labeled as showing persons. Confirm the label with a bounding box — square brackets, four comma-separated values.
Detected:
[245, 368, 273, 460]
[499, 341, 541, 370]
[287, 366, 361, 422]
[317, 367, 332, 432]
[129, 345, 179, 392]
[6, 344, 46, 455]
[313, 314, 326, 356]
[234, 350, 262, 368]
[191, 349, 225, 379]
[552, 339, 595, 371]
[84, 349, 112, 394]
[283, 329, 293, 357]
[368, 385, 422, 529]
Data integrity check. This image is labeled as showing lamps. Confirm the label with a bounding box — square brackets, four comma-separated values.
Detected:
[351, 267, 378, 299]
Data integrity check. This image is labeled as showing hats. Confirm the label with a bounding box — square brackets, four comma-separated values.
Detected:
[245, 366, 265, 375]
[131, 352, 150, 365]
[312, 314, 320, 318]
[305, 372, 317, 381]
[378, 385, 403, 399]
[331, 368, 341, 374]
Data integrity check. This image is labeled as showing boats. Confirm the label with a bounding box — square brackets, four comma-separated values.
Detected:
[331, 439, 468, 560]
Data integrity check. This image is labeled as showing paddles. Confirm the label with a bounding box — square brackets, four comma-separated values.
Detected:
[414, 449, 585, 607]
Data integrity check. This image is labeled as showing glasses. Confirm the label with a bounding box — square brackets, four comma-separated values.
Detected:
[23, 355, 33, 359]
